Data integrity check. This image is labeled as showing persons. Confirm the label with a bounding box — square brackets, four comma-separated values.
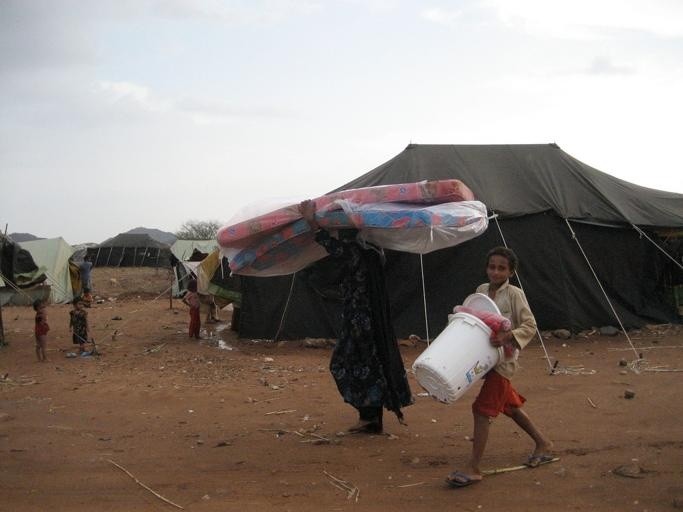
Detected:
[31, 299, 50, 365]
[67, 295, 89, 356]
[198, 291, 226, 324]
[295, 197, 415, 434]
[79, 254, 94, 302]
[67, 256, 81, 303]
[180, 279, 202, 341]
[443, 244, 554, 489]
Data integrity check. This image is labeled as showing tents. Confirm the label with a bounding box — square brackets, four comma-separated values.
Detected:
[229, 142, 682, 341]
[86, 226, 179, 268]
[0, 236, 86, 306]
[168, 238, 220, 262]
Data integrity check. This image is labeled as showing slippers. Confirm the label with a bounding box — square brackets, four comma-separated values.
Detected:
[524, 450, 559, 467]
[445, 470, 479, 486]
[349, 418, 379, 431]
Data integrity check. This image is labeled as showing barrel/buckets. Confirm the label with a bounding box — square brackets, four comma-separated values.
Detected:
[412, 311, 504, 405]
